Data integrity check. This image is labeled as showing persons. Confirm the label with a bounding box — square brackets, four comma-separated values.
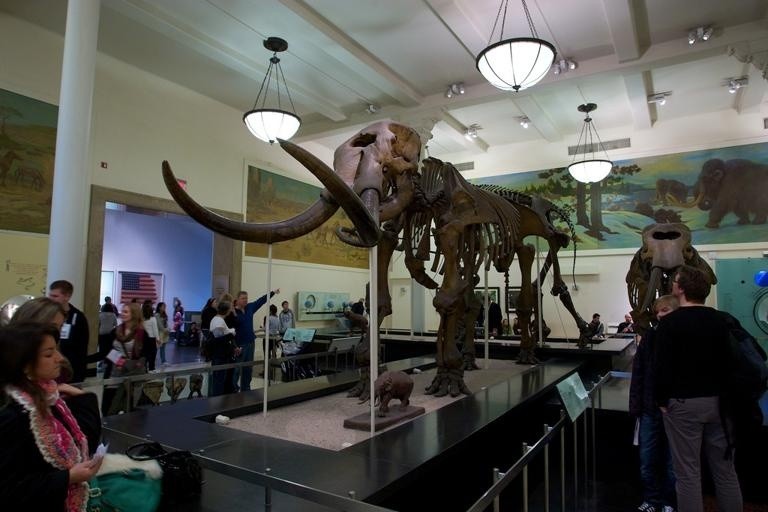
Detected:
[629, 295, 683, 512]
[589, 313, 605, 339]
[650, 265, 746, 512]
[342, 301, 368, 337]
[617, 313, 634, 333]
[479, 296, 504, 336]
[501, 319, 513, 336]
[513, 318, 522, 335]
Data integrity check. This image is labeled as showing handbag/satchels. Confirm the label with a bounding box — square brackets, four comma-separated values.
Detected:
[718, 308, 768, 404]
[124, 440, 203, 512]
[82, 451, 164, 512]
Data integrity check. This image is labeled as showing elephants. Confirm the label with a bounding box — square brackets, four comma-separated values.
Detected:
[358, 370, 414, 417]
[344, 311, 368, 336]
[649, 179, 687, 203]
[665, 158, 768, 228]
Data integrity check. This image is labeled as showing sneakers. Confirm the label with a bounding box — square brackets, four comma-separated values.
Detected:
[661, 504, 674, 512]
[637, 500, 657, 512]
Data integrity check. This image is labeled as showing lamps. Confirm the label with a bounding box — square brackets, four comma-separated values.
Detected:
[474, 1, 554, 92]
[554, 56, 577, 77]
[243, 33, 300, 147]
[446, 81, 467, 99]
[648, 22, 749, 108]
[466, 124, 481, 139]
[568, 100, 613, 183]
[517, 114, 531, 130]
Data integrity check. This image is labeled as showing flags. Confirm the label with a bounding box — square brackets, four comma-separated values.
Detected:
[119, 274, 160, 305]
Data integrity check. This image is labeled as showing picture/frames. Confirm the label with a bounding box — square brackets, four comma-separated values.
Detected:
[473, 287, 499, 305]
[241, 157, 372, 275]
[504, 285, 534, 314]
[117, 269, 164, 314]
